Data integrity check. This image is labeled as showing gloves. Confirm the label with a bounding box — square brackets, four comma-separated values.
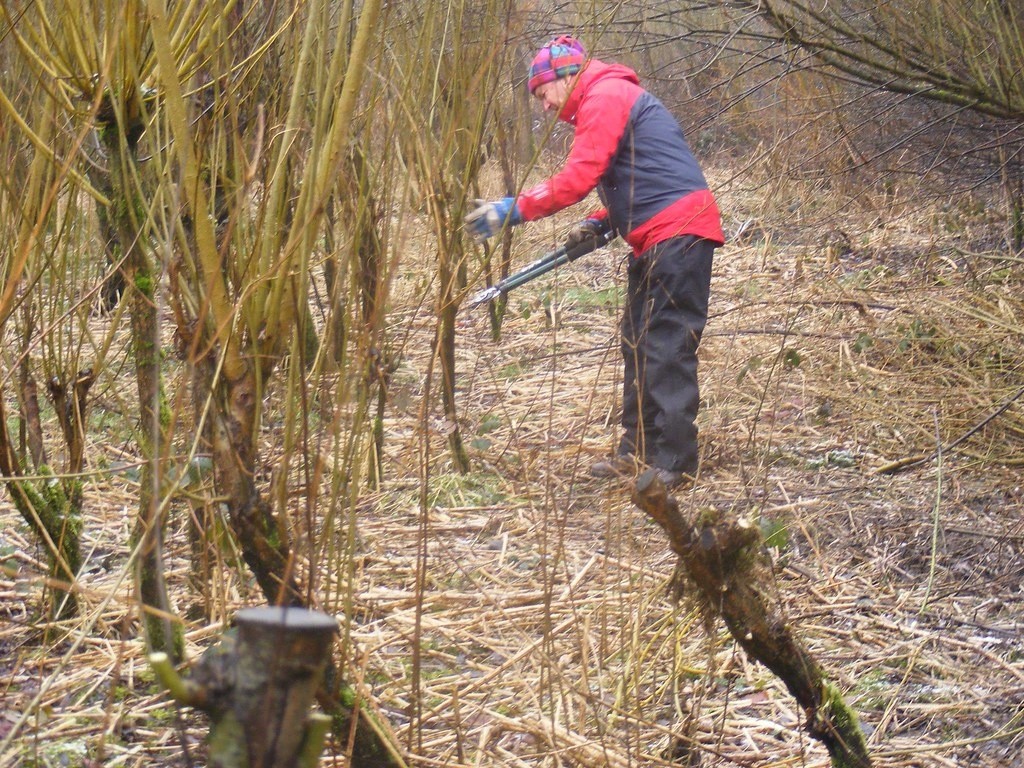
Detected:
[567, 220, 604, 246]
[464, 197, 520, 244]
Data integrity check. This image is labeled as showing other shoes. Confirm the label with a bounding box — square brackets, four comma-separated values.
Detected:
[653, 466, 684, 487]
[593, 454, 644, 475]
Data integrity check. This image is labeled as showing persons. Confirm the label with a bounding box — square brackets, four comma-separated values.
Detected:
[463, 33, 725, 489]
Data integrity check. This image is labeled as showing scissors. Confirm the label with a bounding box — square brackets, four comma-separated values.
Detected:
[454, 232, 608, 316]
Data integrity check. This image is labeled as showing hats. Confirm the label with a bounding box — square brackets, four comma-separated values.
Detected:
[528, 33, 588, 93]
